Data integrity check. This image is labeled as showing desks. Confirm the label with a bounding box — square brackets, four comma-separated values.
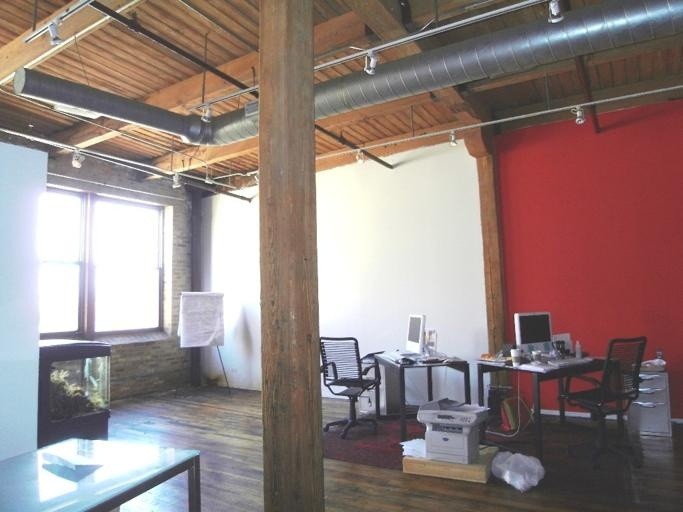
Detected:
[2, 436, 205, 512]
[374, 351, 608, 465]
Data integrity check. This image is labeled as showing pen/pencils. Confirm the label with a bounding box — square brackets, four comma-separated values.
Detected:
[551, 341, 566, 352]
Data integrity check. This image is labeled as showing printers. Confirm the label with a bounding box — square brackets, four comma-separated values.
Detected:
[417, 406, 489, 464]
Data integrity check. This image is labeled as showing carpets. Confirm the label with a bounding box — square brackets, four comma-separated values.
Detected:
[318, 413, 622, 493]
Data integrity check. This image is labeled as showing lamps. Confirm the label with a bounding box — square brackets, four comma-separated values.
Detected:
[200, 105, 214, 124]
[46, 22, 64, 46]
[355, 151, 365, 165]
[547, 0, 564, 24]
[71, 151, 85, 169]
[447, 130, 458, 147]
[571, 106, 586, 126]
[364, 53, 377, 77]
[171, 173, 183, 189]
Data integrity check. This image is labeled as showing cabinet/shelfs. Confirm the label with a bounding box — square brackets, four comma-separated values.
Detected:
[626, 370, 673, 437]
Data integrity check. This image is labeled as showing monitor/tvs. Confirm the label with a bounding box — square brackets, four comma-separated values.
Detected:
[513, 311, 551, 355]
[407, 315, 428, 354]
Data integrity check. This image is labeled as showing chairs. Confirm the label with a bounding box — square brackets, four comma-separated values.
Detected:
[315, 336, 381, 441]
[558, 337, 647, 463]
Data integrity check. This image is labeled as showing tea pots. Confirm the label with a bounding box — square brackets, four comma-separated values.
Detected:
[551, 341, 566, 361]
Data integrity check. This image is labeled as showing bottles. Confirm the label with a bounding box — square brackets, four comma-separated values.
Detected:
[576, 341, 581, 359]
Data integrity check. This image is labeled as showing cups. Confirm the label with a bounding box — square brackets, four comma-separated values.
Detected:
[510, 349, 522, 367]
[532, 350, 541, 361]
